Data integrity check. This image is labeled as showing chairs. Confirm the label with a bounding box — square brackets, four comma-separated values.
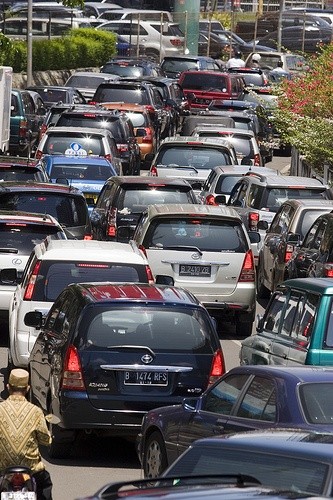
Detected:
[152, 313, 175, 340]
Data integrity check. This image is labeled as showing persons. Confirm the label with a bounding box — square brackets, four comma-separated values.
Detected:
[227, 51, 246, 69]
[273, 60, 286, 73]
[249, 53, 261, 68]
[0, 368, 52, 500]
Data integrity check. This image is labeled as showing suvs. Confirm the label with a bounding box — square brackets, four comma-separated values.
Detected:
[117, 203, 262, 339]
[256, 198, 333, 301]
[213, 171, 333, 267]
[191, 165, 288, 206]
[86, 175, 204, 244]
[0, 155, 70, 201]
[23, 279, 228, 461]
[0, 182, 101, 240]
[0, 234, 175, 386]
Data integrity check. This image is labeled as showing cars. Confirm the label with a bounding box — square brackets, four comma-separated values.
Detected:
[137, 364, 333, 482]
[0, 210, 69, 311]
[40, 154, 119, 219]
[1, 0, 333, 203]
[283, 209, 333, 279]
[73, 421, 333, 500]
[239, 277, 333, 368]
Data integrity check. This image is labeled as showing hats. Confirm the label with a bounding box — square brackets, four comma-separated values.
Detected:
[9, 369, 30, 388]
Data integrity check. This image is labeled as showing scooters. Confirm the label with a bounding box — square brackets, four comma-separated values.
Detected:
[0, 411, 62, 500]
